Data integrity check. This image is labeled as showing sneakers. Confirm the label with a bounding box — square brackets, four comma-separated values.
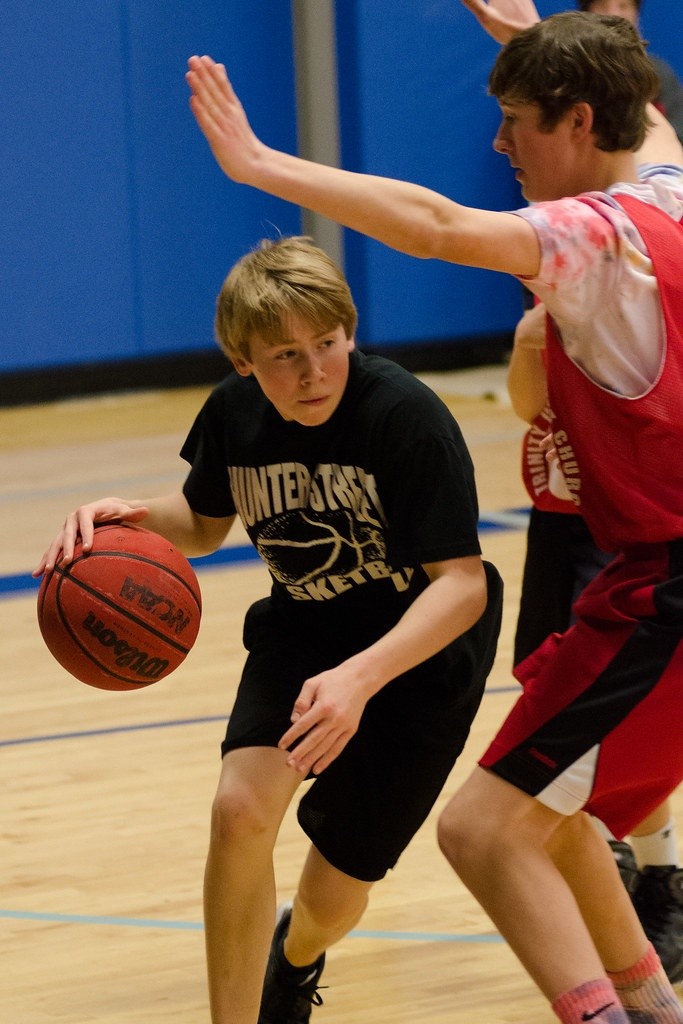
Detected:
[259, 900, 329, 1024]
[609, 841, 637, 899]
[636, 863, 683, 982]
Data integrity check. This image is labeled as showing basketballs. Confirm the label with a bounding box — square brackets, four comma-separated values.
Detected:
[35, 522, 202, 692]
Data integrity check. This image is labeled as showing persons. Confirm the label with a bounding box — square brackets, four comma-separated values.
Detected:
[184, 0, 682, 1023]
[33, 238, 504, 1024]
